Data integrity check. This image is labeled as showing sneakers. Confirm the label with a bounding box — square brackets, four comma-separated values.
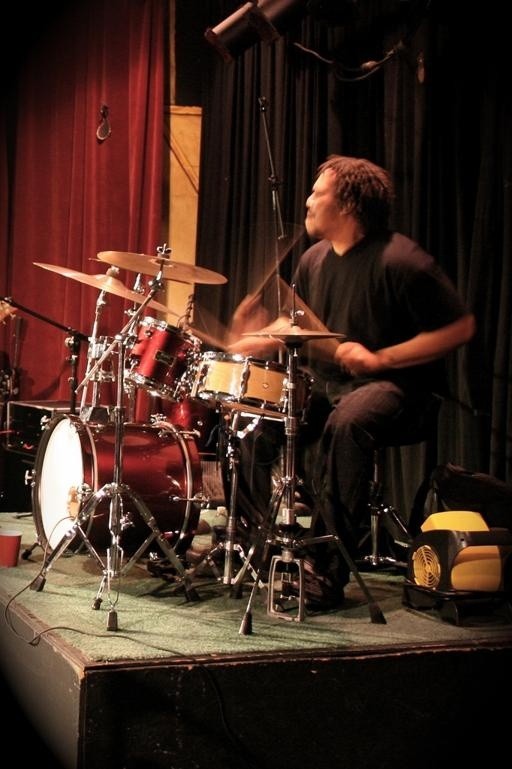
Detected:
[213, 546, 258, 581]
[282, 576, 345, 610]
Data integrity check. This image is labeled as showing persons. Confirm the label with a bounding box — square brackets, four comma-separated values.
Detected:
[199, 157, 473, 615]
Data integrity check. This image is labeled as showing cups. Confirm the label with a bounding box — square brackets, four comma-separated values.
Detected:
[2, 529, 22, 569]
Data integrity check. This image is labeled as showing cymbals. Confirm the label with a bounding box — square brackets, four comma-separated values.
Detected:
[241, 325, 347, 343]
[32, 261, 183, 320]
[97, 251, 228, 284]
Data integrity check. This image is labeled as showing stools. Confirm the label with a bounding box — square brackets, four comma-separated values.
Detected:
[353, 417, 438, 576]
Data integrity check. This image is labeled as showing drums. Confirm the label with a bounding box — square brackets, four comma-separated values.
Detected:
[123, 315, 203, 405]
[185, 350, 317, 425]
[25, 412, 211, 564]
